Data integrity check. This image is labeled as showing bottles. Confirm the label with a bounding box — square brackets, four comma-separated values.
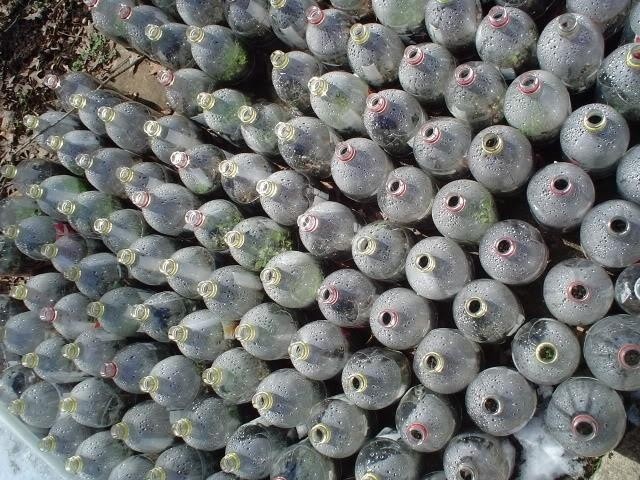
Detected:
[0, 0, 640, 480]
